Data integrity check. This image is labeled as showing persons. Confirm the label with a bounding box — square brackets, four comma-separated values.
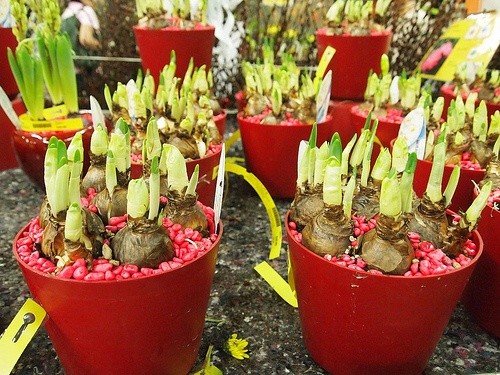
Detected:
[50, 0, 105, 100]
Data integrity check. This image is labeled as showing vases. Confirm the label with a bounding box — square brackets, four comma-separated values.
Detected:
[0, 23, 28, 101]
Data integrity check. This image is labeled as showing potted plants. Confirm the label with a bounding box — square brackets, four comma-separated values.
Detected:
[160, 48, 227, 143]
[351, 54, 432, 174]
[235, 34, 335, 201]
[91, 68, 222, 217]
[132, 0, 215, 99]
[471, 133, 500, 339]
[407, 83, 500, 209]
[285, 106, 493, 375]
[13, 116, 225, 375]
[440, 63, 500, 130]
[4, 29, 112, 190]
[314, 0, 392, 100]
